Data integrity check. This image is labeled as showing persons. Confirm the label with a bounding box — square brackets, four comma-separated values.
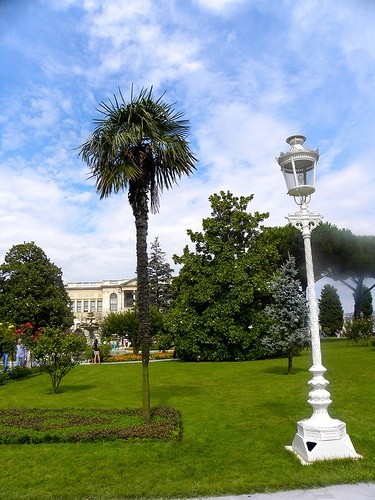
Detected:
[3, 353, 8, 371]
[92, 339, 101, 364]
[33, 359, 37, 366]
[15, 339, 27, 367]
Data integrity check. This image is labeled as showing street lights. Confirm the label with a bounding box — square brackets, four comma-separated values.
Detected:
[274, 136, 362, 466]
